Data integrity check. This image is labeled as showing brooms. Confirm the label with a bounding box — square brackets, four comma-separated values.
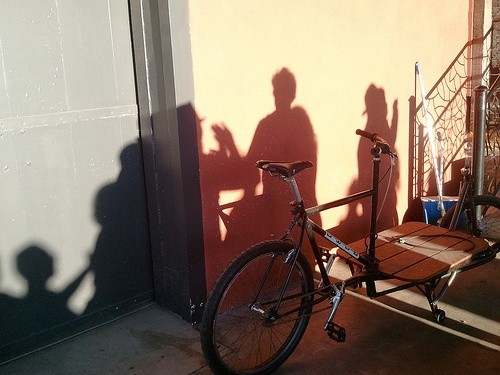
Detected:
[413, 64, 461, 225]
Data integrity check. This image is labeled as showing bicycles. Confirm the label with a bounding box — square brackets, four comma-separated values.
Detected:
[200, 128, 500, 374]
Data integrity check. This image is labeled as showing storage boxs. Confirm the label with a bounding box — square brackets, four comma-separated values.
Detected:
[419, 194, 468, 228]
